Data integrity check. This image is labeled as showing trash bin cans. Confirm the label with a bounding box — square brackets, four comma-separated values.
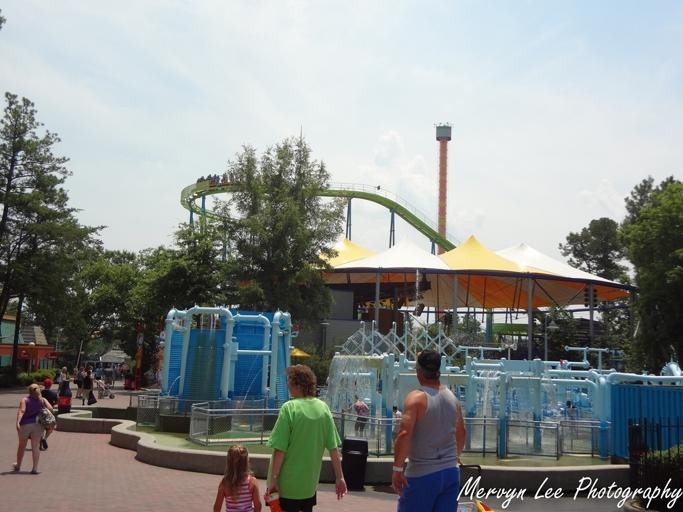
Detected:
[341, 438, 368, 492]
[124, 375, 133, 390]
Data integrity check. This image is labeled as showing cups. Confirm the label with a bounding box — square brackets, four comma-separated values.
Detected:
[265, 491, 281, 512]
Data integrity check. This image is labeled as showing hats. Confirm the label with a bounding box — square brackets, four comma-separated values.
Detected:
[418, 350, 441, 369]
[44, 379, 54, 385]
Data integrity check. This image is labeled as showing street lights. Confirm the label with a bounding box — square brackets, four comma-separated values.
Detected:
[28, 341, 35, 375]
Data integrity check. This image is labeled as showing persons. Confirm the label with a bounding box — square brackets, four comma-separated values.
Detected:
[351, 394, 369, 437]
[73, 361, 130, 406]
[262, 364, 349, 511]
[56, 366, 72, 391]
[391, 405, 401, 441]
[566, 398, 576, 432]
[391, 347, 466, 511]
[10, 382, 53, 475]
[213, 443, 261, 512]
[39, 377, 58, 450]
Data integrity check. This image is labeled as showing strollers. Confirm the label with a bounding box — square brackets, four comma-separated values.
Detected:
[94, 380, 115, 399]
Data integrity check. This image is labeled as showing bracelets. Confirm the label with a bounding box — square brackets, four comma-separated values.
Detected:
[271, 471, 281, 477]
[392, 464, 403, 472]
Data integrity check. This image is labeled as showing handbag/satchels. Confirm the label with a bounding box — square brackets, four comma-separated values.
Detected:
[88, 391, 96, 405]
[40, 408, 55, 428]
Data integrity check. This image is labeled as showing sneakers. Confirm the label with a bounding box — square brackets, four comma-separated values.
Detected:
[43, 439, 48, 449]
